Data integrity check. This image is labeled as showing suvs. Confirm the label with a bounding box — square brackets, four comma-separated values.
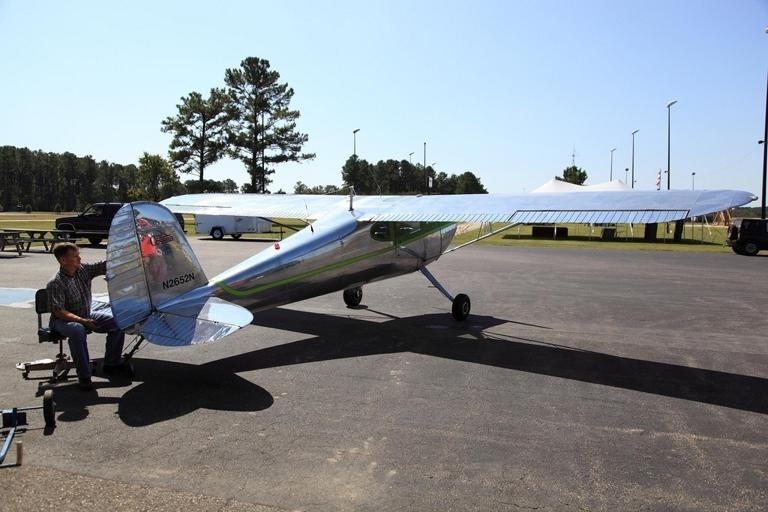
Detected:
[725, 217, 768, 257]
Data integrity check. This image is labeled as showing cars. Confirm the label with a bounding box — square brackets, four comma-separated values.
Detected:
[55, 202, 185, 246]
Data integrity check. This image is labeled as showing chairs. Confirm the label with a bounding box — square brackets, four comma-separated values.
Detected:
[22, 287, 98, 393]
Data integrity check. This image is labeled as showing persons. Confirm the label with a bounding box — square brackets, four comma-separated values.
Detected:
[46, 242, 125, 397]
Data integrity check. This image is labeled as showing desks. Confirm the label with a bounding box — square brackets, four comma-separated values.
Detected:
[0, 227, 82, 255]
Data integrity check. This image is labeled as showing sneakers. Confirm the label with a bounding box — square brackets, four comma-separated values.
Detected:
[78, 381, 97, 400]
[104, 362, 133, 376]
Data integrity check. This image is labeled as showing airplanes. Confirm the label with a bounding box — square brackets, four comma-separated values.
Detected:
[91, 184, 759, 374]
